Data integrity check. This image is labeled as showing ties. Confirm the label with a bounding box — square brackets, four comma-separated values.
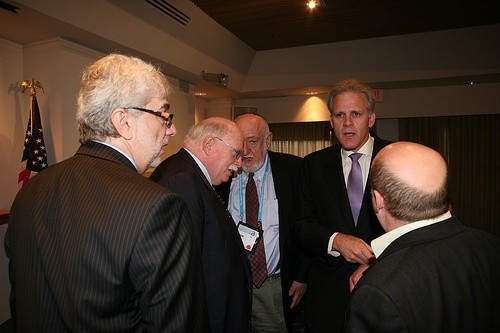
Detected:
[346, 153, 364, 228]
[211, 184, 237, 231]
[245, 171, 269, 289]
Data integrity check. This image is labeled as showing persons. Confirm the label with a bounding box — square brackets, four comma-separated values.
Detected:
[347, 142, 500, 333]
[148, 118, 253, 333]
[292, 80, 394, 333]
[210, 114, 306, 333]
[4, 54, 208, 333]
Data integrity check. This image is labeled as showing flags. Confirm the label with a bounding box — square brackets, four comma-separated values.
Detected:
[17, 94, 48, 191]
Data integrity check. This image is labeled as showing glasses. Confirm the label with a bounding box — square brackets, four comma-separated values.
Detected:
[124, 104, 175, 129]
[214, 136, 243, 161]
